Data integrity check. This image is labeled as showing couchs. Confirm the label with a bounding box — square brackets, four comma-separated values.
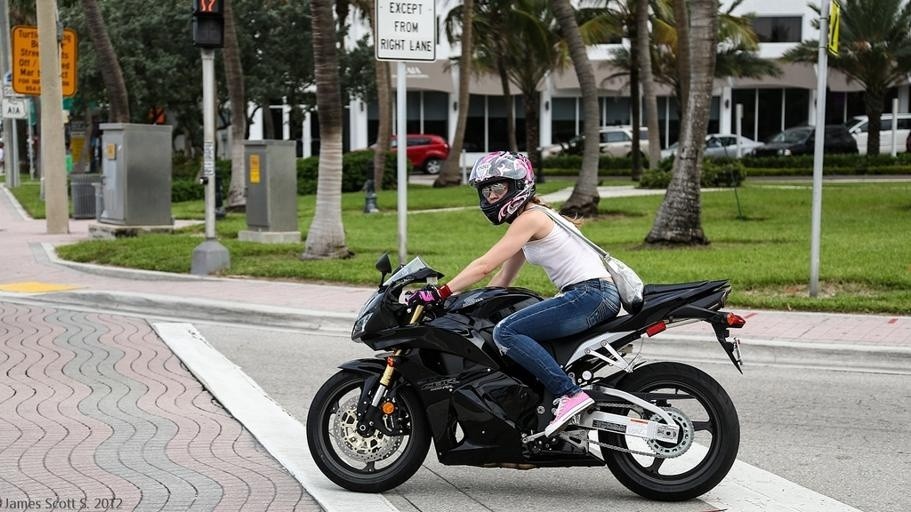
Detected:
[191, 0, 225, 50]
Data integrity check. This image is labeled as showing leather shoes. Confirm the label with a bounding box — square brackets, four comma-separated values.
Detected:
[406, 284, 442, 308]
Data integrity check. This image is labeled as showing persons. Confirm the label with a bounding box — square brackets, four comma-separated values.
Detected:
[405, 151, 621, 439]
[0, 142, 6, 174]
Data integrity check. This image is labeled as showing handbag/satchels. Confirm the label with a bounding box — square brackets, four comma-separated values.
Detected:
[436, 284, 452, 299]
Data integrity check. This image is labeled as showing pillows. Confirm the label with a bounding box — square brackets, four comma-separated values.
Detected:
[467, 152, 537, 225]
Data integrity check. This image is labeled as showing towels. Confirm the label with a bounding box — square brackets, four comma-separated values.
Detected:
[67, 173, 100, 219]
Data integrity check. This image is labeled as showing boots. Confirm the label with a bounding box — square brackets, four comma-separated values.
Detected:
[752, 124, 862, 159]
[368, 130, 451, 176]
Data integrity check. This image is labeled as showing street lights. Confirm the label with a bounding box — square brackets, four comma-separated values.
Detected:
[603, 255, 644, 314]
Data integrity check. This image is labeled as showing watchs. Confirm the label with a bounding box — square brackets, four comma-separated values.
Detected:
[306, 249, 748, 503]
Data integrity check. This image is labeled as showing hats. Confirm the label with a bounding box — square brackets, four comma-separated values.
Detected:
[663, 130, 766, 165]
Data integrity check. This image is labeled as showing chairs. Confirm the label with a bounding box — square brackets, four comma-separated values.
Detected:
[544, 390, 595, 438]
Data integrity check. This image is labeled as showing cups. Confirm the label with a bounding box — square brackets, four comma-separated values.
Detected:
[481, 184, 509, 197]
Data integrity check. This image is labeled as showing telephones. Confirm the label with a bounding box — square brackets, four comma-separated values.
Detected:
[373, 0, 440, 63]
[1, 18, 82, 131]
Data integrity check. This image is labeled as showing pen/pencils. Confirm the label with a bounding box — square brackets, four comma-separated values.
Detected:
[844, 113, 911, 159]
[539, 122, 655, 168]
